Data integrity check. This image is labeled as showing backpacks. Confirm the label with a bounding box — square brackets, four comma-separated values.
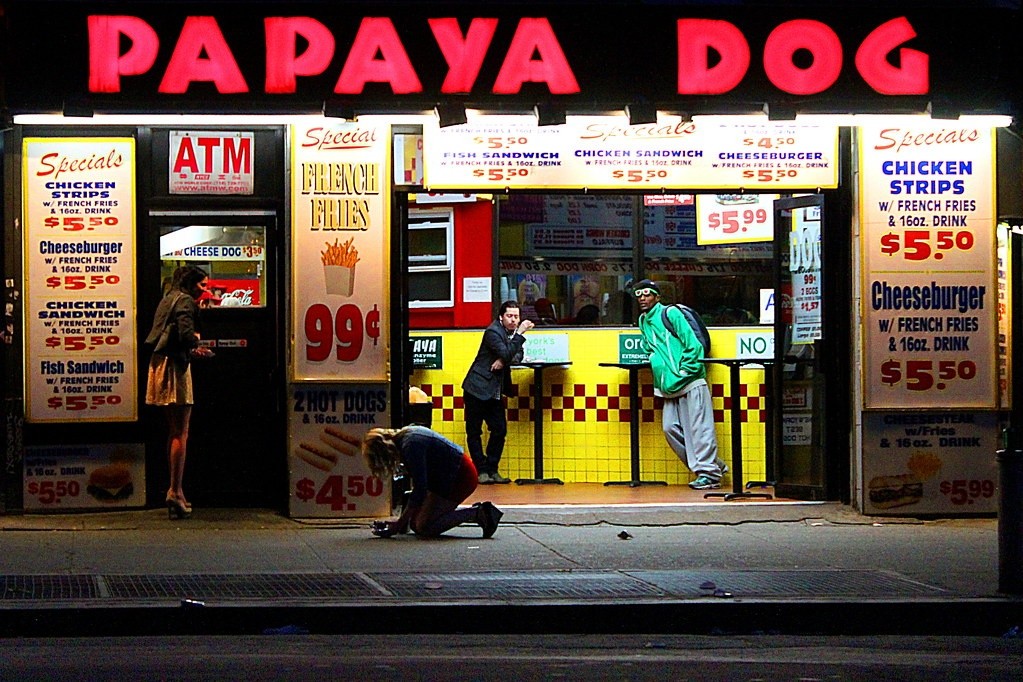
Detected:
[641, 304, 711, 356]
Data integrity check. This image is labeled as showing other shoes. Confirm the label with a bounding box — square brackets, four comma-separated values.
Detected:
[478, 473, 494, 484]
[489, 472, 511, 483]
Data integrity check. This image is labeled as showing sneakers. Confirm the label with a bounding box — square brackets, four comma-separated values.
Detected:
[687, 476, 721, 489]
[720, 464, 729, 474]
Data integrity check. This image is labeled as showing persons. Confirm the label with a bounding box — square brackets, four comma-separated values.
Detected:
[461, 300, 535, 485]
[629, 277, 730, 490]
[143, 265, 215, 518]
[361, 424, 505, 539]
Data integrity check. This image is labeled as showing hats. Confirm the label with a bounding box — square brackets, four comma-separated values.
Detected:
[632, 279, 660, 294]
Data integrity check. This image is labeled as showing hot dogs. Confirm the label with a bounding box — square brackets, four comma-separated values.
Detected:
[868, 474, 924, 509]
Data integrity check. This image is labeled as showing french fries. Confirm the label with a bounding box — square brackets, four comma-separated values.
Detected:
[906, 451, 942, 481]
[320, 237, 361, 268]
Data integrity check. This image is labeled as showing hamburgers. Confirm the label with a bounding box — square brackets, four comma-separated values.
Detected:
[86, 465, 134, 504]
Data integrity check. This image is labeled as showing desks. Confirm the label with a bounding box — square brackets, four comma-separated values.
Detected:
[698, 357, 775, 500]
[509, 361, 574, 486]
[598, 362, 669, 488]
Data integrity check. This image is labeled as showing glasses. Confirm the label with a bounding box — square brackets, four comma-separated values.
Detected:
[635, 287, 659, 296]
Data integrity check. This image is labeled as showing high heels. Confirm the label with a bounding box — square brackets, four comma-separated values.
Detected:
[167, 487, 191, 508]
[166, 494, 192, 519]
[472, 501, 504, 539]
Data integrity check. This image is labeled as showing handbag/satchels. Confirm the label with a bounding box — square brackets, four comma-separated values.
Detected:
[153, 294, 195, 371]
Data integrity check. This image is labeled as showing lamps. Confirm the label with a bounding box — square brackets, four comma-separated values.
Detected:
[625, 102, 657, 125]
[529, 101, 566, 127]
[434, 99, 467, 128]
[321, 98, 355, 120]
[925, 100, 961, 120]
[761, 101, 797, 121]
[63, 100, 94, 118]
